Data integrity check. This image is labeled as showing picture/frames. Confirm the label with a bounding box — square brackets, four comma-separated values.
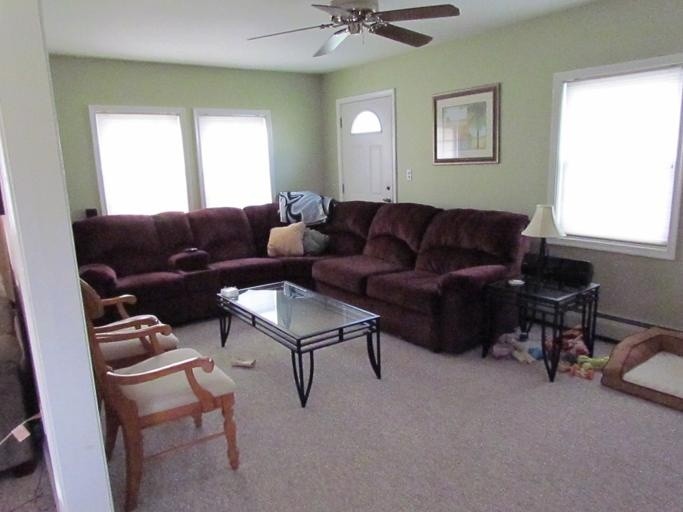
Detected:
[431, 82, 502, 165]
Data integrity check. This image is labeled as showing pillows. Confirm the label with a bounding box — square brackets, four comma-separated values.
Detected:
[169, 246, 208, 269]
[303, 227, 331, 257]
[267, 220, 306, 257]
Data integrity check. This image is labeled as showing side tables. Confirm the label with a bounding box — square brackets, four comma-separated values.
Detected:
[506, 276, 600, 382]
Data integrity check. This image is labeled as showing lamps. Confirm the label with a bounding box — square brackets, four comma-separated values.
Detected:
[521, 203, 565, 282]
[331, 8, 378, 35]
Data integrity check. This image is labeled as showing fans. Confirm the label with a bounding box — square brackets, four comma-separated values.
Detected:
[247, 1, 461, 56]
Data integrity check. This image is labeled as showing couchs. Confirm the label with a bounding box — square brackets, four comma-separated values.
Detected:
[75, 203, 529, 358]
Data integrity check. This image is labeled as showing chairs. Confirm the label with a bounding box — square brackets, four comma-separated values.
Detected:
[86, 324, 240, 512]
[78, 277, 180, 414]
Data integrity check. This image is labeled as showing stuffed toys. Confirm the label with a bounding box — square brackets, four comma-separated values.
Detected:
[492, 325, 610, 380]
